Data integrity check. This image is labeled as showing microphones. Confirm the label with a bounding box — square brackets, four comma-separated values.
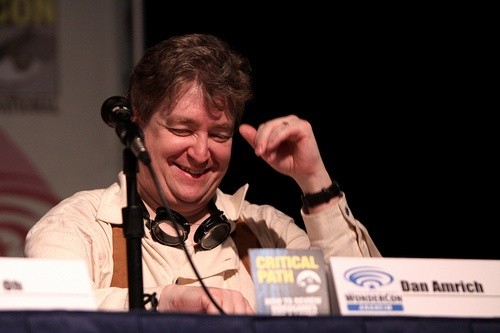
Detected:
[102, 96, 152, 165]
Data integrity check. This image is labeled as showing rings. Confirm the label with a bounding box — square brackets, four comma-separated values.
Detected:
[284, 120, 290, 127]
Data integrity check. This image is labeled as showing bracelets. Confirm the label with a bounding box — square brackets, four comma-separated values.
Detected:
[301, 181, 342, 210]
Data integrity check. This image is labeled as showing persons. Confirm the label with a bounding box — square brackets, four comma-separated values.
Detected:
[23, 35, 382, 317]
